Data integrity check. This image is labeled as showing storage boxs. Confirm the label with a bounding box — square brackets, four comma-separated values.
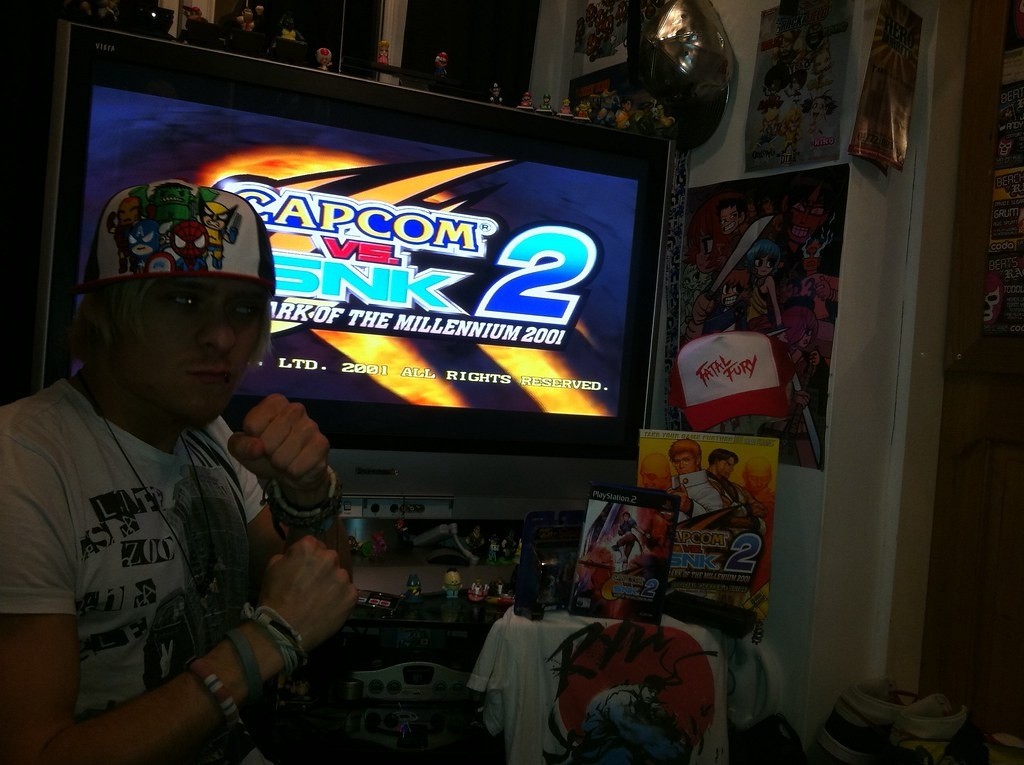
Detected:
[568, 481, 682, 626]
[512, 510, 585, 620]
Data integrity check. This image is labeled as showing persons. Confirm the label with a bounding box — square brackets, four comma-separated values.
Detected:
[488, 82, 502, 104]
[575, 100, 592, 118]
[560, 99, 571, 113]
[539, 94, 552, 109]
[521, 92, 532, 107]
[378, 40, 388, 64]
[440, 568, 463, 598]
[0, 177, 358, 765]
[316, 48, 333, 70]
[436, 52, 448, 78]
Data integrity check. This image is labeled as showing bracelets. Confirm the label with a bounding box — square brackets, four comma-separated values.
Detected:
[185, 655, 239, 727]
[238, 602, 306, 677]
[260, 464, 343, 541]
[224, 627, 263, 696]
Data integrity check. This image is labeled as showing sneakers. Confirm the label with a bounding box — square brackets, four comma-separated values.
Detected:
[816, 678, 1024, 765]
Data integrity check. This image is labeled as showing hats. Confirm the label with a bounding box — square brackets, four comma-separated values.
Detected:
[667, 330, 795, 430]
[85, 178, 275, 292]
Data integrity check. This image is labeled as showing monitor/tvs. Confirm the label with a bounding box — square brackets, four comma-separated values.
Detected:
[32, 15, 673, 518]
